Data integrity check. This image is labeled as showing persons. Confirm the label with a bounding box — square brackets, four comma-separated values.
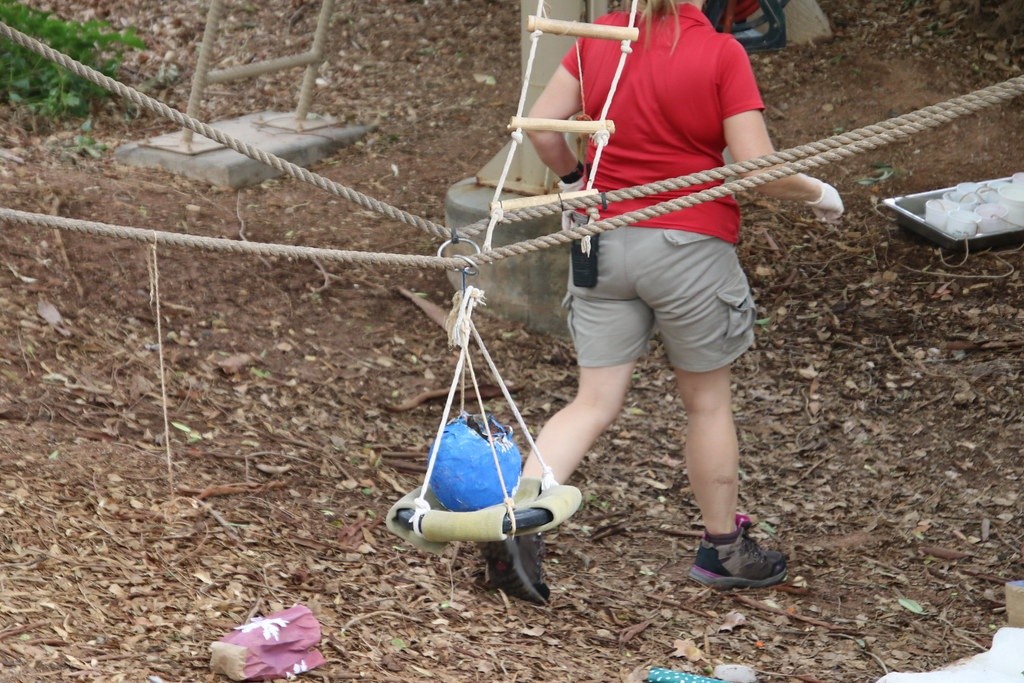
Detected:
[484, 0, 844, 608]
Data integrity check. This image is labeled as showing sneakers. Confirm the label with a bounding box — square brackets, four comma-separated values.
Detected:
[687, 512, 786, 591]
[477, 532, 550, 605]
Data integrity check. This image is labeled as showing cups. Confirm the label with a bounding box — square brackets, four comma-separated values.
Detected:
[924, 171, 1024, 238]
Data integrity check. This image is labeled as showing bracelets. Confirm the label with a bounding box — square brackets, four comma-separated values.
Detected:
[559, 160, 584, 185]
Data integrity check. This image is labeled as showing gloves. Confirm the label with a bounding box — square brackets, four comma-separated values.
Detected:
[802, 177, 844, 221]
[557, 177, 585, 231]
